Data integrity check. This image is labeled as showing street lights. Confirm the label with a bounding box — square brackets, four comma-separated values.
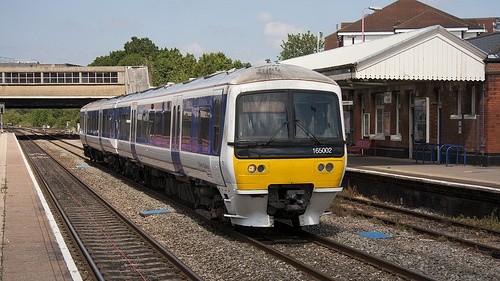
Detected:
[361, 6, 383, 42]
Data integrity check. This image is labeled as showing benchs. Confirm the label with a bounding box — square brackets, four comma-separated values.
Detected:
[346, 140, 377, 160]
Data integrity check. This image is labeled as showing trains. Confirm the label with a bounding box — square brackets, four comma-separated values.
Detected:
[80, 62, 348, 229]
